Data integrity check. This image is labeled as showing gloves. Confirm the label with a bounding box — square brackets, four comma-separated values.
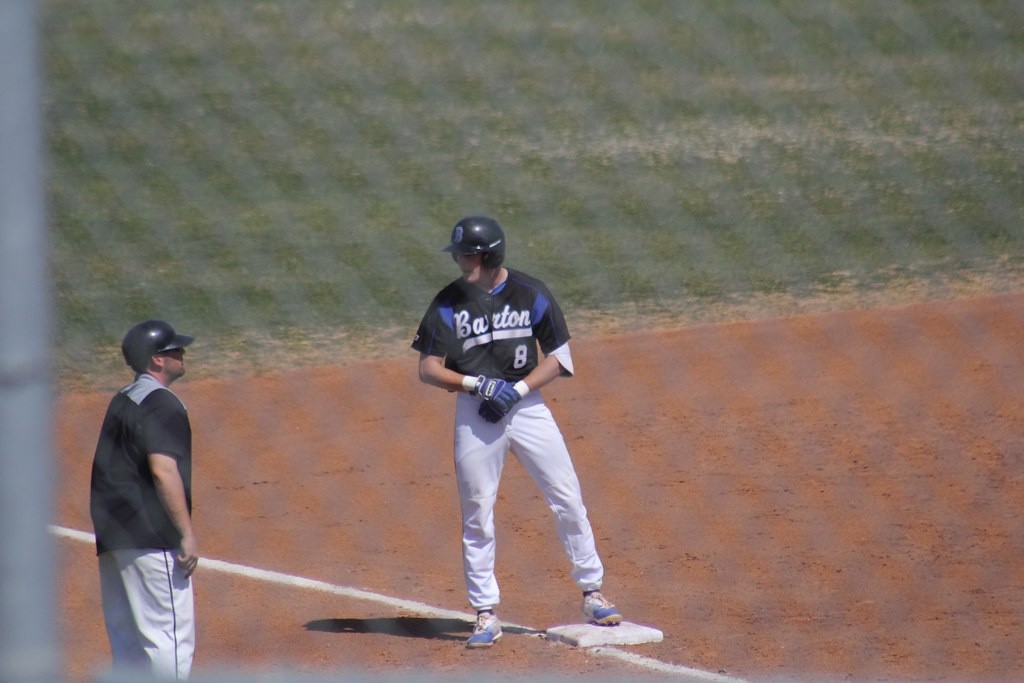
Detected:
[475, 375, 521, 424]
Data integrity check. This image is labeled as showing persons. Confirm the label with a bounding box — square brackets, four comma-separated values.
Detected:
[91, 320, 199, 683]
[410, 217, 622, 648]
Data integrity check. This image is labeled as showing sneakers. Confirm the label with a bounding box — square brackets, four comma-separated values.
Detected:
[465, 612, 502, 647]
[581, 592, 623, 625]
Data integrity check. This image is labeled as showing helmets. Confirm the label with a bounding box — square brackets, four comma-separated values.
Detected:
[441, 216, 505, 269]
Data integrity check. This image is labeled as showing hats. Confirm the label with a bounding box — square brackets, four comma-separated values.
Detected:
[122, 319, 194, 372]
